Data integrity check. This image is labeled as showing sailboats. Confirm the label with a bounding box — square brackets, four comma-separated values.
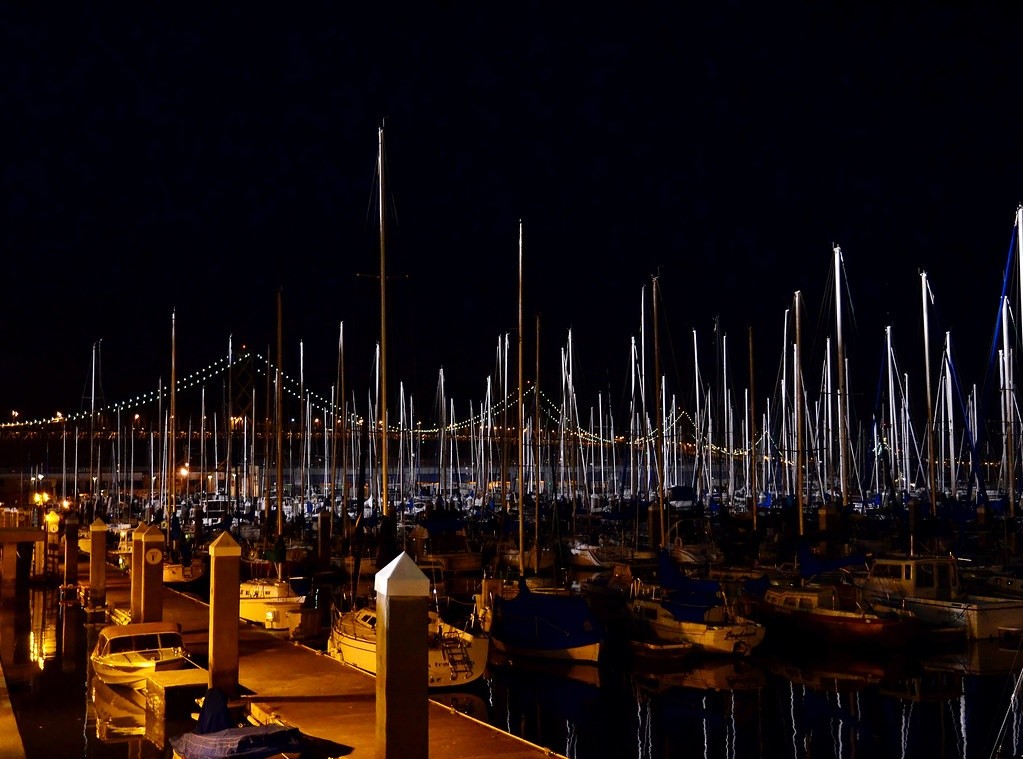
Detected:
[1, 122, 1021, 688]
